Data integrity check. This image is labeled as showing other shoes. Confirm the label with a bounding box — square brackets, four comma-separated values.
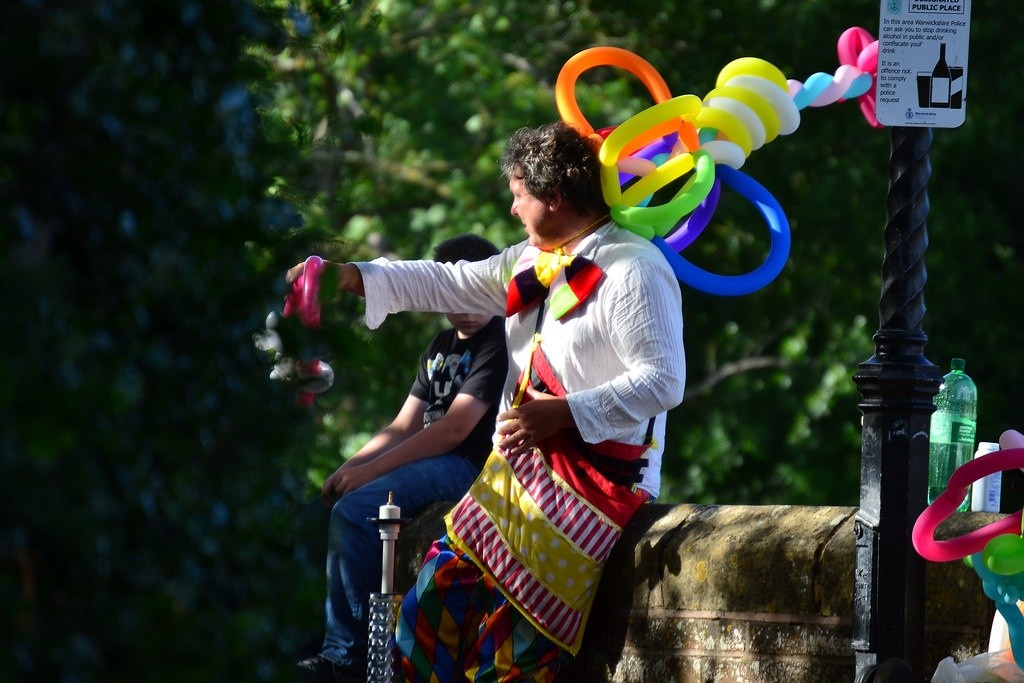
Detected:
[297, 655, 366, 683]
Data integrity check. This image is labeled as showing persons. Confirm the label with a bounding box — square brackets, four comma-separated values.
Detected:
[283, 122, 686, 683]
[289, 235, 509, 680]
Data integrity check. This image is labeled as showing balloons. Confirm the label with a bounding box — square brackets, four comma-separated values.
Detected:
[251, 254, 334, 404]
[912, 429, 1024, 669]
[554, 24, 887, 296]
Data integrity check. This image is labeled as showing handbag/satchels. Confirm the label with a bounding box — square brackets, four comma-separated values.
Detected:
[441, 338, 660, 660]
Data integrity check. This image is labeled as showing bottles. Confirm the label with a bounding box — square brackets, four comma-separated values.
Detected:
[927, 358, 977, 512]
[971, 441, 1002, 513]
